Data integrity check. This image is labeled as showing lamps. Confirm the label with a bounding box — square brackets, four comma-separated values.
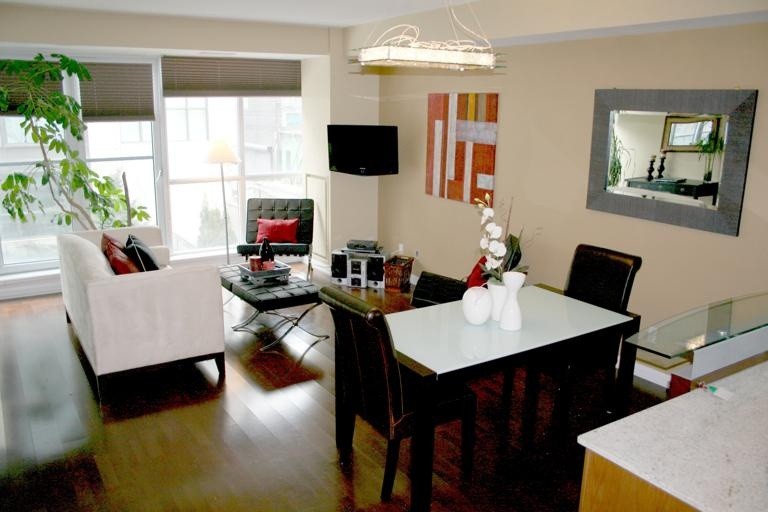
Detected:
[356, 0, 496, 69]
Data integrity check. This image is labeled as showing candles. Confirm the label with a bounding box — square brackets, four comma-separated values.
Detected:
[660, 154, 666, 159]
[649, 155, 656, 162]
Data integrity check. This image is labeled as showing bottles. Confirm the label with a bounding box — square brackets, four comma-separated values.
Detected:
[259, 236, 274, 261]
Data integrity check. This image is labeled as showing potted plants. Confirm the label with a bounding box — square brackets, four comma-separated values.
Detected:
[695, 129, 724, 184]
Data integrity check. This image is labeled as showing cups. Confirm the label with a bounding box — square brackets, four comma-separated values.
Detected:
[462, 272, 526, 332]
[249, 256, 262, 272]
[262, 261, 275, 271]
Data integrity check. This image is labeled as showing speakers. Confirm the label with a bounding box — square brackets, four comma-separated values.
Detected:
[332, 255, 347, 278]
[367, 257, 383, 281]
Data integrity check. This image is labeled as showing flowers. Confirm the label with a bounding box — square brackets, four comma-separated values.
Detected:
[474, 192, 530, 280]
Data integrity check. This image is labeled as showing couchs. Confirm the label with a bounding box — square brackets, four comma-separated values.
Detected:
[56, 225, 228, 405]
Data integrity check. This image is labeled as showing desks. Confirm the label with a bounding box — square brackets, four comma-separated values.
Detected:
[624, 176, 719, 207]
[382, 284, 639, 501]
[574, 289, 768, 512]
[329, 248, 390, 290]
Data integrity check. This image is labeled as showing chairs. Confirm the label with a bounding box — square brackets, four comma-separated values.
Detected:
[318, 285, 478, 502]
[233, 198, 315, 279]
[409, 234, 521, 308]
[520, 244, 642, 455]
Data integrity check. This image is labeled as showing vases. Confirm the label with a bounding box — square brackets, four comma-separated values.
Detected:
[500, 270, 525, 330]
[484, 276, 506, 322]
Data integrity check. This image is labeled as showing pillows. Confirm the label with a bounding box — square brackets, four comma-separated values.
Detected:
[255, 218, 299, 244]
[102, 230, 138, 275]
[125, 234, 160, 272]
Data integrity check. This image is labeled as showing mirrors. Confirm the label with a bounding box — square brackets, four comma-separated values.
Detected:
[586, 88, 759, 238]
[659, 114, 720, 152]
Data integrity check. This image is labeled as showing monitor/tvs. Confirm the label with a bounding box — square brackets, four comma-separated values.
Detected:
[327, 125, 398, 176]
